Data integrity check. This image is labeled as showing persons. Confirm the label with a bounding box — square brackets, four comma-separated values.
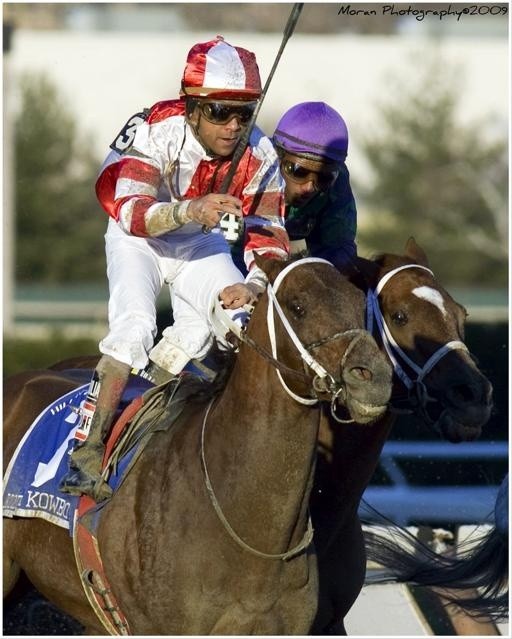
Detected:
[268, 101, 379, 289]
[61, 36, 292, 500]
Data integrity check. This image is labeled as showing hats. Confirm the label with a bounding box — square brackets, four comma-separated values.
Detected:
[179, 40, 262, 100]
[273, 102, 348, 164]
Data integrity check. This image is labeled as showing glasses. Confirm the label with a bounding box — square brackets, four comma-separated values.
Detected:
[199, 101, 258, 125]
[282, 160, 340, 192]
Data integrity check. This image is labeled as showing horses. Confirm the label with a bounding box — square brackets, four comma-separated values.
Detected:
[64, 235, 508, 635]
[3, 247, 396, 638]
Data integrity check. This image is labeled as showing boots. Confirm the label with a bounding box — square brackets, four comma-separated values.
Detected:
[139, 357, 176, 386]
[57, 354, 133, 498]
